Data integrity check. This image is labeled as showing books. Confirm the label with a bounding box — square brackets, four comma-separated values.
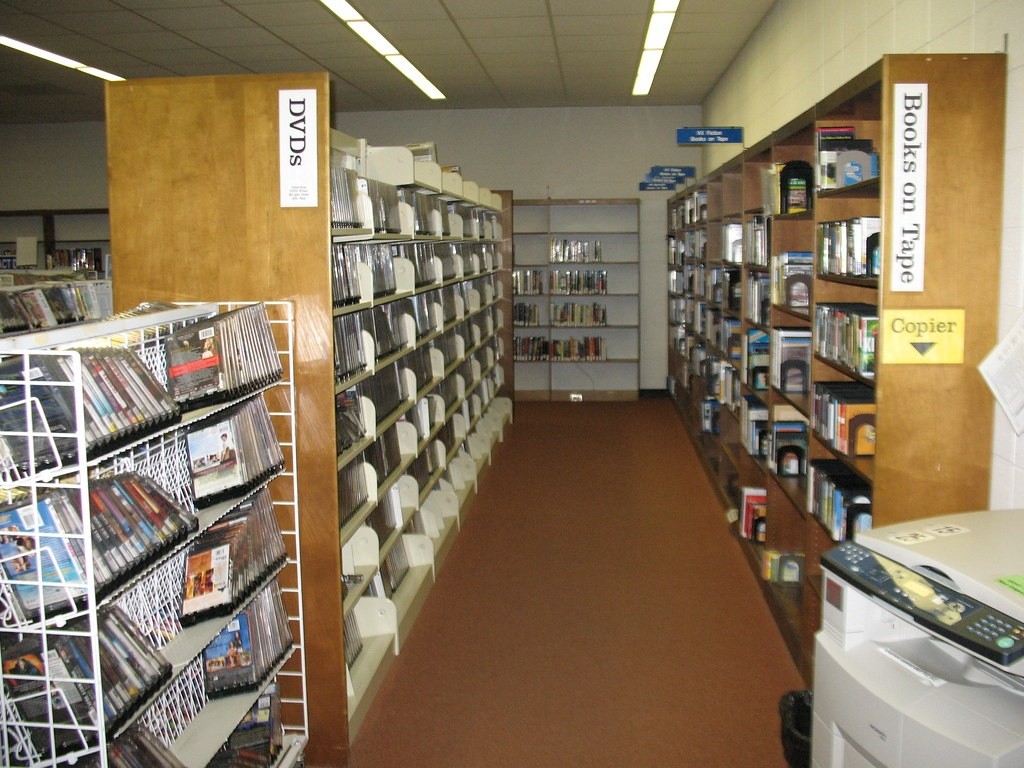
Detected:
[550, 268, 607, 294]
[770, 325, 812, 394]
[816, 215, 880, 280]
[811, 381, 875, 459]
[513, 336, 549, 362]
[551, 238, 601, 263]
[769, 405, 811, 477]
[770, 250, 815, 311]
[550, 335, 607, 361]
[666, 160, 770, 478]
[770, 163, 815, 217]
[762, 547, 805, 582]
[549, 302, 607, 327]
[0, 246, 112, 335]
[514, 303, 539, 326]
[813, 127, 878, 194]
[512, 270, 542, 295]
[738, 486, 766, 543]
[814, 301, 879, 377]
[807, 459, 871, 542]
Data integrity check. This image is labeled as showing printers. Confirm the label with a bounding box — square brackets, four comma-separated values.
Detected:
[812, 508, 1024, 768]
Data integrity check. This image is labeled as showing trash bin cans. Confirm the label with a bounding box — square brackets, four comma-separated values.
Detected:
[784, 690, 811, 768]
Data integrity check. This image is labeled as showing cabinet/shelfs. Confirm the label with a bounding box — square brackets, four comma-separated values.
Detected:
[1, 52, 1006, 768]
